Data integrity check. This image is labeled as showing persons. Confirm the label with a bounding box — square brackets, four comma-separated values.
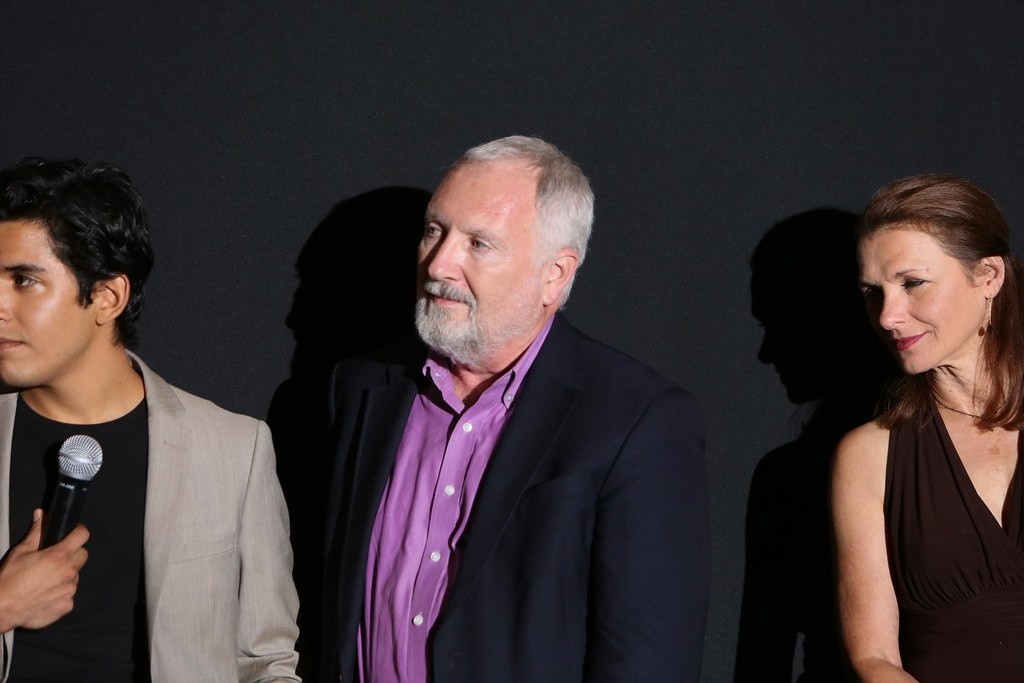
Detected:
[829, 173, 1024, 683]
[0, 158, 307, 683]
[295, 131, 710, 683]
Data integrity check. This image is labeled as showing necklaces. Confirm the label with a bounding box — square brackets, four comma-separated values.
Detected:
[928, 383, 1013, 432]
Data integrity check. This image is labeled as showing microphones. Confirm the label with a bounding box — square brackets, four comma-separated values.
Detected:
[36, 435, 103, 553]
